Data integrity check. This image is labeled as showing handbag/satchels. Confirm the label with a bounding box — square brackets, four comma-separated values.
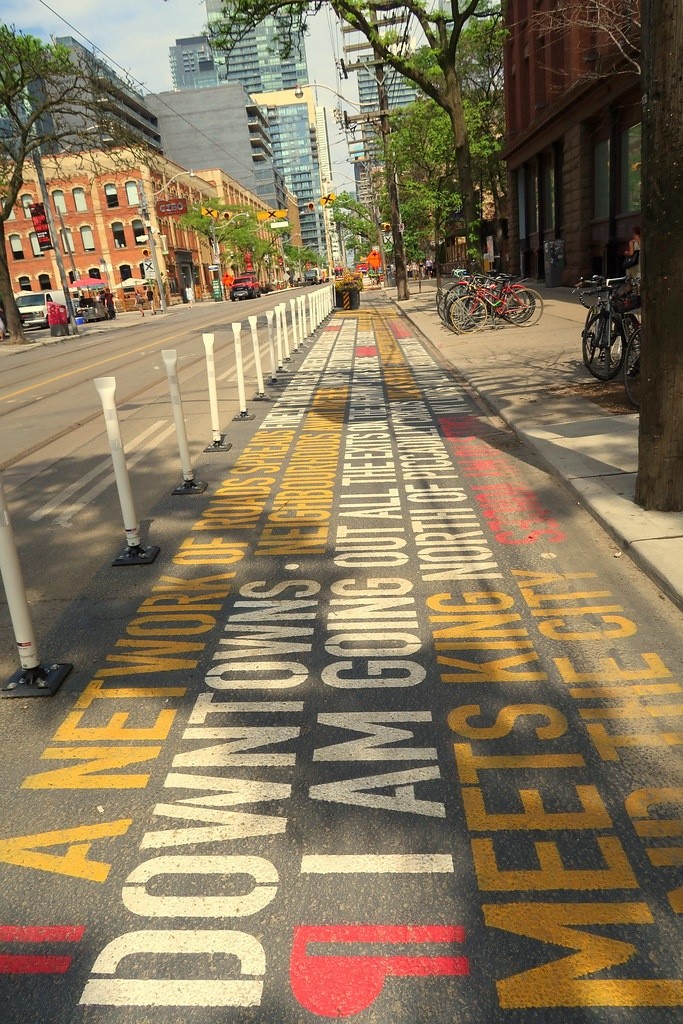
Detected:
[139, 298, 145, 304]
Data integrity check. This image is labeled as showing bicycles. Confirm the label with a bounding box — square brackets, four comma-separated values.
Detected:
[436, 270, 545, 334]
[571, 275, 644, 409]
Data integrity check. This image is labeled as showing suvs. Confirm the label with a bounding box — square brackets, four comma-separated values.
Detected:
[231, 276, 261, 302]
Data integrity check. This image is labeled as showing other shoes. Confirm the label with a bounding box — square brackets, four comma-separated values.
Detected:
[429, 277, 431, 279]
[151, 312, 156, 315]
[142, 313, 144, 317]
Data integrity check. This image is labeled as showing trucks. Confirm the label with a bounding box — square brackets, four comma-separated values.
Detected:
[16, 290, 70, 327]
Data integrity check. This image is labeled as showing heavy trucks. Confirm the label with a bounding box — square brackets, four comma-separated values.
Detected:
[304, 268, 327, 285]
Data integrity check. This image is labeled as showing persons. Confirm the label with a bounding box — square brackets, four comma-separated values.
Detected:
[159, 289, 166, 314]
[624, 231, 641, 296]
[79, 288, 116, 320]
[147, 286, 156, 315]
[406, 257, 433, 280]
[368, 264, 396, 279]
[133, 290, 145, 317]
[185, 285, 195, 309]
[0, 300, 7, 340]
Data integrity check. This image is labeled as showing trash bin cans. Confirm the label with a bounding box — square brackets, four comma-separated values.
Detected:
[387, 273, 397, 287]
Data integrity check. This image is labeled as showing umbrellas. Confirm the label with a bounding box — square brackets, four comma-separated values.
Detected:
[68, 278, 106, 298]
[110, 278, 148, 290]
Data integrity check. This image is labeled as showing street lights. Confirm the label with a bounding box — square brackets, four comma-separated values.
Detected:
[294, 82, 409, 301]
[33, 124, 116, 335]
[322, 170, 390, 289]
[211, 209, 249, 302]
[142, 169, 196, 315]
